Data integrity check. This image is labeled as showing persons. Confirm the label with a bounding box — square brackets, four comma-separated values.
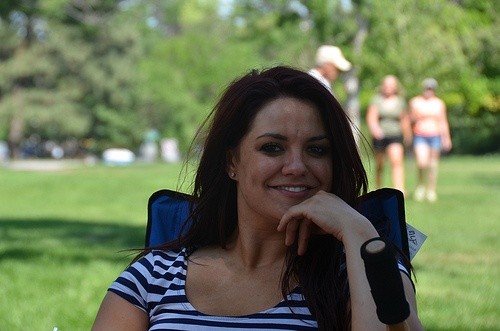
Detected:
[366, 74, 415, 199]
[90, 64, 426, 331]
[305, 44, 354, 92]
[408, 76, 453, 202]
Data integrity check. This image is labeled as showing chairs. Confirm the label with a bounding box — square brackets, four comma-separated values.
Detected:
[145, 189, 410, 269]
